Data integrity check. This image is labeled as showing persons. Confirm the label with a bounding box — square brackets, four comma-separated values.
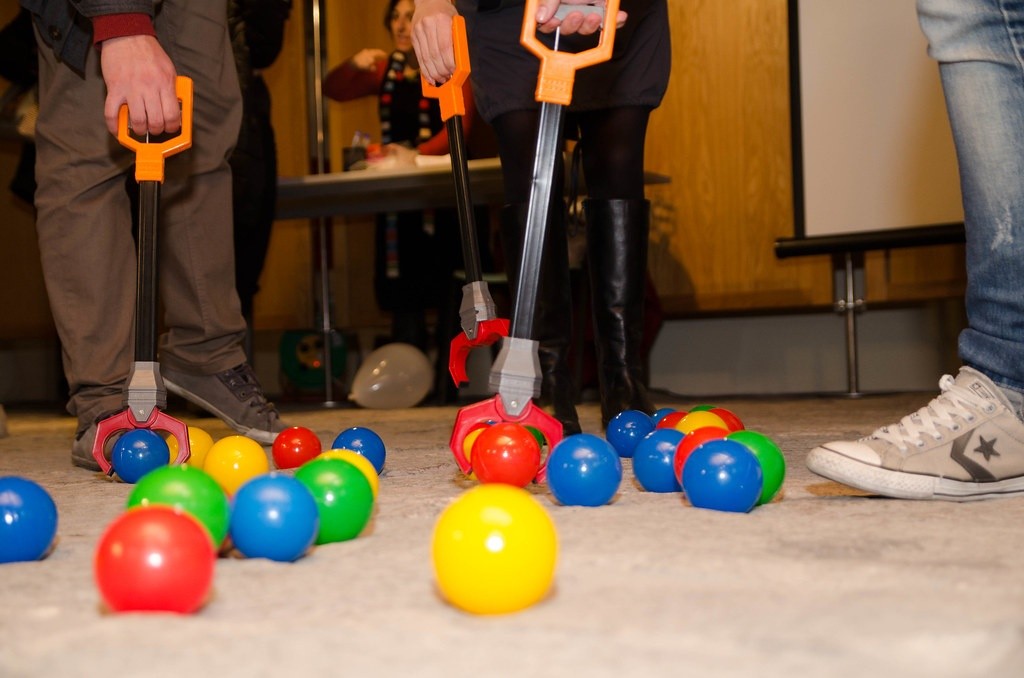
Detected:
[321, 0, 478, 404]
[410, 1, 671, 446]
[0, 0, 293, 470]
[804, 0, 1024, 503]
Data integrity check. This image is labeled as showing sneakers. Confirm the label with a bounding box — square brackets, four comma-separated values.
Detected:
[806, 366, 1024, 503]
[71, 407, 127, 471]
[157, 353, 288, 443]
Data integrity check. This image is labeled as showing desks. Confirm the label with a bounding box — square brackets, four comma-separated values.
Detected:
[274, 159, 671, 410]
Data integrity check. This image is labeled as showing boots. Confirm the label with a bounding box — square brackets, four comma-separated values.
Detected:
[583, 199, 658, 429]
[500, 200, 582, 445]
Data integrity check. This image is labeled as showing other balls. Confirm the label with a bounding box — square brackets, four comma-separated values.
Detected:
[98, 507, 214, 618]
[110, 421, 387, 563]
[449, 409, 551, 485]
[0, 477, 58, 565]
[429, 483, 559, 619]
[548, 398, 784, 510]
